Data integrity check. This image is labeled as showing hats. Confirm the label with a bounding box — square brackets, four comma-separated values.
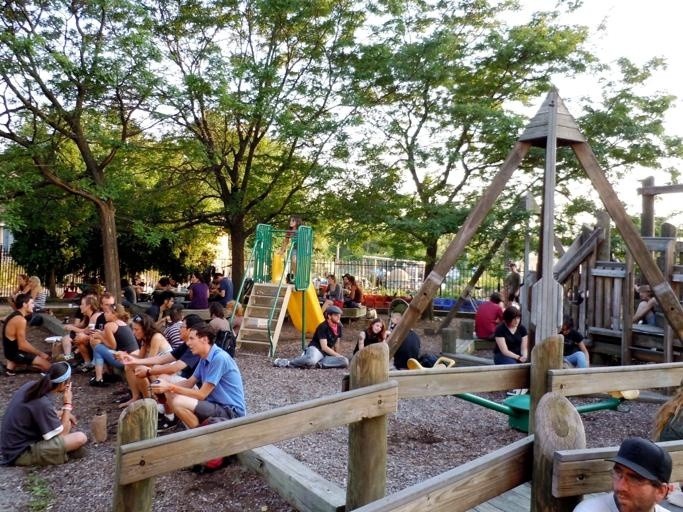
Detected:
[326, 306, 343, 315]
[605, 436, 671, 486]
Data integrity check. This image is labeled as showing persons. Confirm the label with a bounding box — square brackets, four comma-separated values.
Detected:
[475, 292, 504, 340]
[504, 264, 524, 306]
[321, 273, 363, 313]
[634, 285, 659, 325]
[574, 436, 672, 512]
[1, 361, 88, 467]
[392, 313, 421, 369]
[2, 272, 243, 432]
[353, 319, 386, 354]
[273, 306, 349, 369]
[494, 307, 528, 364]
[560, 313, 590, 367]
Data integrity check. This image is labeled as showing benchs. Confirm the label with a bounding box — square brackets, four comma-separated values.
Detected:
[180, 308, 230, 321]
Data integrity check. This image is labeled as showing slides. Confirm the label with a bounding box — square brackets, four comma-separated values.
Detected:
[272, 248, 327, 332]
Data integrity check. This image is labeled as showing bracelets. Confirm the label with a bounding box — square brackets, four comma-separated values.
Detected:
[62, 407, 72, 411]
[518, 356, 521, 360]
[64, 402, 70, 404]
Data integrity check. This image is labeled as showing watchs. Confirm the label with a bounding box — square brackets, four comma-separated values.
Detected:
[146, 369, 151, 376]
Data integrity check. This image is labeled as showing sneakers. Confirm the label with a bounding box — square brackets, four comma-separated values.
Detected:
[66, 362, 177, 458]
[274, 358, 289, 367]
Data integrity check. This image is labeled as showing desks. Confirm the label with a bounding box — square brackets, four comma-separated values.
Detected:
[135, 288, 214, 309]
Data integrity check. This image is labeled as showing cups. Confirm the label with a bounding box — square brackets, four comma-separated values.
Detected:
[88, 324, 95, 331]
[68, 303, 72, 308]
[63, 316, 70, 324]
[74, 318, 80, 324]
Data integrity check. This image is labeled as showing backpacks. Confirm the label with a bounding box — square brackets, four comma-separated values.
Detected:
[190, 417, 232, 474]
